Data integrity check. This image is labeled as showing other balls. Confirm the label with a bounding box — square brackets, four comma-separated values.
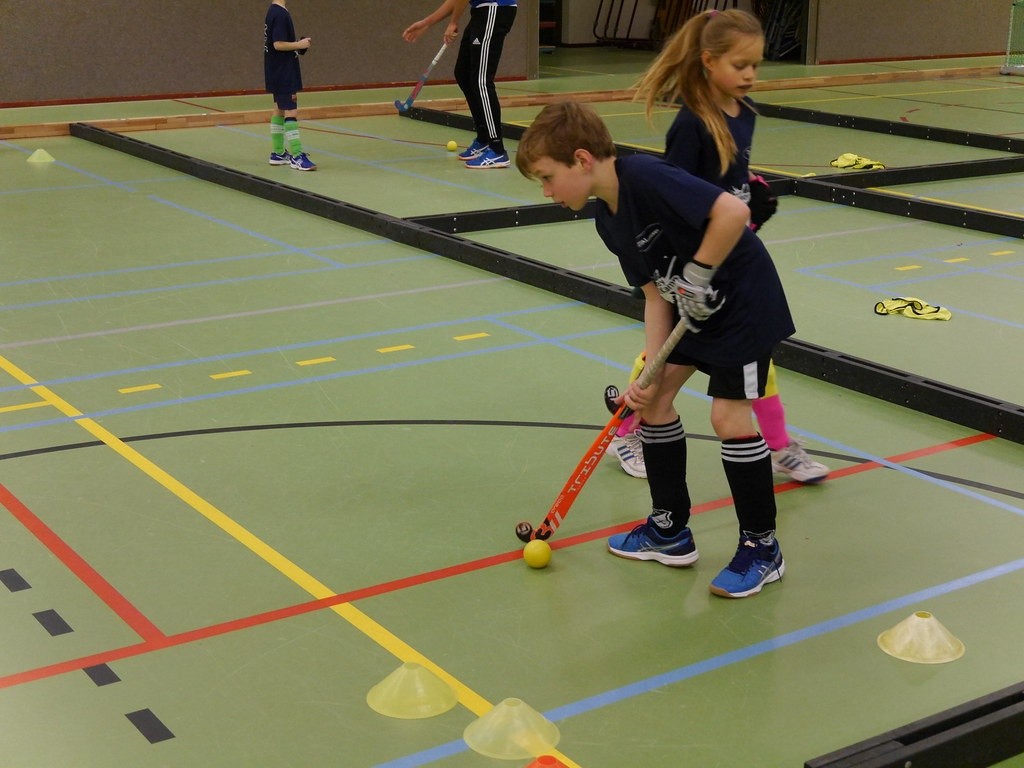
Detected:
[522, 538, 553, 570]
[446, 140, 458, 151]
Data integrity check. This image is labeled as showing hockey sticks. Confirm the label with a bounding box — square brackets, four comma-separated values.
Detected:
[393, 32, 456, 113]
[513, 316, 690, 544]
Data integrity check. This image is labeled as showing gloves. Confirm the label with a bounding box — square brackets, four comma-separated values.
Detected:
[674, 258, 726, 334]
[747, 173, 779, 234]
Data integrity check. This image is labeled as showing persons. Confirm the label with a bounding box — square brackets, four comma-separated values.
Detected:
[516, 102, 795, 600]
[605, 8, 830, 484]
[264, 0, 317, 170]
[402, 0, 519, 168]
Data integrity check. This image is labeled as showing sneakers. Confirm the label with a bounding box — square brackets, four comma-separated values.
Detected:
[464, 147, 511, 169]
[457, 139, 489, 160]
[289, 153, 318, 171]
[770, 439, 830, 483]
[610, 428, 648, 479]
[268, 148, 294, 164]
[708, 530, 786, 598]
[607, 514, 700, 567]
